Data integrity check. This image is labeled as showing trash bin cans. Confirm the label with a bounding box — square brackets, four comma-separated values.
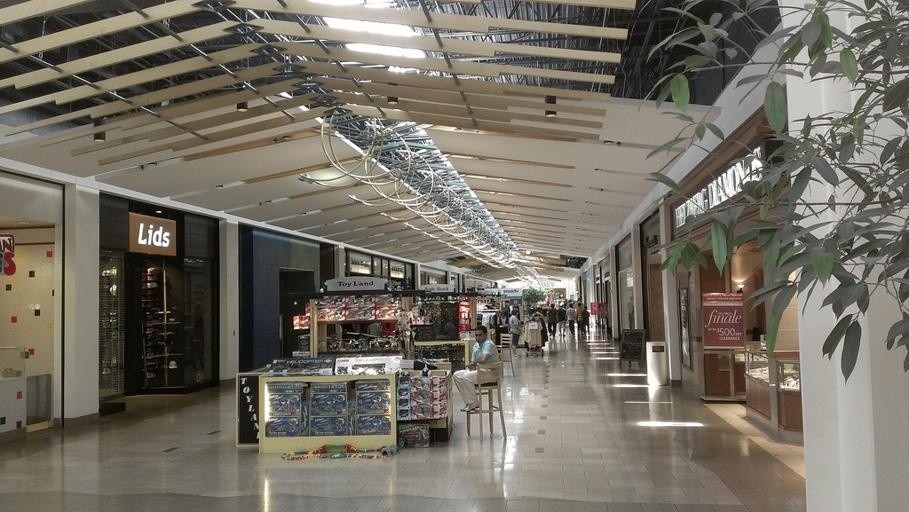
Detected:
[646, 341, 668, 385]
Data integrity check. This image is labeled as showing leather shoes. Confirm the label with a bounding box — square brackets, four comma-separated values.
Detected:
[460, 401, 480, 412]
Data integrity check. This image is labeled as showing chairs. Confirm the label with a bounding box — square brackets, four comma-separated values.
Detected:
[467, 360, 507, 442]
[495, 333, 515, 378]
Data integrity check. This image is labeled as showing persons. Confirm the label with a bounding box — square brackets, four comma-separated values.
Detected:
[544, 298, 591, 337]
[509, 309, 523, 358]
[451, 323, 501, 413]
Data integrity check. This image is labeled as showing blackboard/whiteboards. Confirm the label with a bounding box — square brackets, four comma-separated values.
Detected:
[620, 329, 647, 360]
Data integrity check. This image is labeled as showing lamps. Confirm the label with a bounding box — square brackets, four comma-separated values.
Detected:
[387, 83, 398, 104]
[545, 96, 557, 117]
[95, 118, 105, 143]
[237, 88, 248, 112]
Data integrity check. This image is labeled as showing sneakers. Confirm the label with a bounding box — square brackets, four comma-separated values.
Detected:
[511, 329, 590, 357]
